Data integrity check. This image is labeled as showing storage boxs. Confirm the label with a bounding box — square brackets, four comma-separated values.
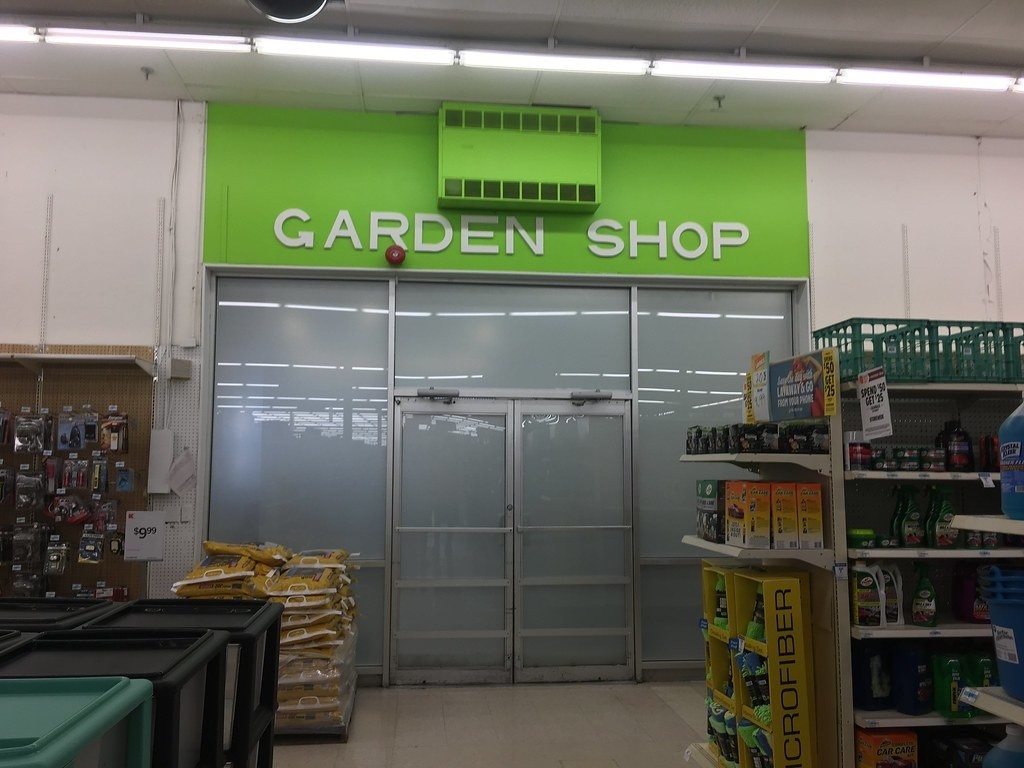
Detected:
[854, 729, 917, 768]
[686, 420, 828, 455]
[813, 317, 1024, 386]
[927, 729, 997, 768]
[696, 480, 823, 550]
[0, 595, 286, 768]
[702, 556, 818, 768]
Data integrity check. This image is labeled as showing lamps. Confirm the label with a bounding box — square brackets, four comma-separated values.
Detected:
[0, 13, 1024, 95]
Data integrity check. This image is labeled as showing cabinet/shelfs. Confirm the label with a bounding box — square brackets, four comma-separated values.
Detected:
[949, 515, 1024, 730]
[680, 349, 1024, 768]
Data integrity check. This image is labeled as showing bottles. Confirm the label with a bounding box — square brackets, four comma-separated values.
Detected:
[983, 724, 1024, 768]
[937, 420, 974, 472]
[999, 390, 1024, 518]
[874, 558, 905, 625]
[851, 561, 886, 628]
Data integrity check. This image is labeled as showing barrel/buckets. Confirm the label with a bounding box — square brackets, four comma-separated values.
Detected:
[980, 565, 1024, 704]
